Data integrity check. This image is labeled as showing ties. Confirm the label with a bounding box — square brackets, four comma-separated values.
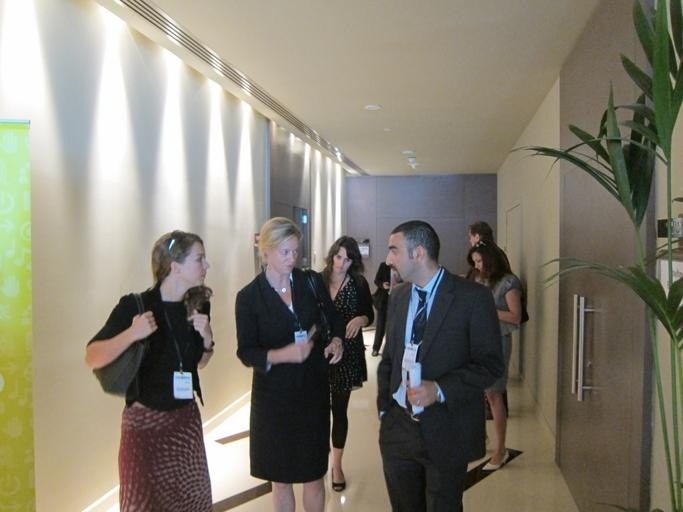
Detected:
[405, 287, 427, 418]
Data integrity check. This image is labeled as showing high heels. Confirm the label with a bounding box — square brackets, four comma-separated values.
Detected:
[481, 450, 509, 471]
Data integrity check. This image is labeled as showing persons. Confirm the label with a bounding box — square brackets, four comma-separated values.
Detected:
[85, 230, 215, 511]
[377, 219, 500, 511]
[235, 215, 347, 512]
[319, 236, 375, 490]
[464, 223, 512, 281]
[467, 242, 522, 471]
[371, 255, 404, 356]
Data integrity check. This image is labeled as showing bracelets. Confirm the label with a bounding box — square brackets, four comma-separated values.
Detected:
[203, 340, 214, 353]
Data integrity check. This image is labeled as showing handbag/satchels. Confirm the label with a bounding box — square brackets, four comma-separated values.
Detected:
[312, 312, 350, 373]
[93, 342, 144, 394]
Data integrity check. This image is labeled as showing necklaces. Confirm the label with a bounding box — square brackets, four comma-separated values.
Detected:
[275, 284, 291, 293]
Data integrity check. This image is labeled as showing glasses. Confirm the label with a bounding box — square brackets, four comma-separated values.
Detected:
[168, 229, 186, 251]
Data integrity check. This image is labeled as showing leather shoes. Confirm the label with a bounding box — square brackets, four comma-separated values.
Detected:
[332, 468, 346, 492]
